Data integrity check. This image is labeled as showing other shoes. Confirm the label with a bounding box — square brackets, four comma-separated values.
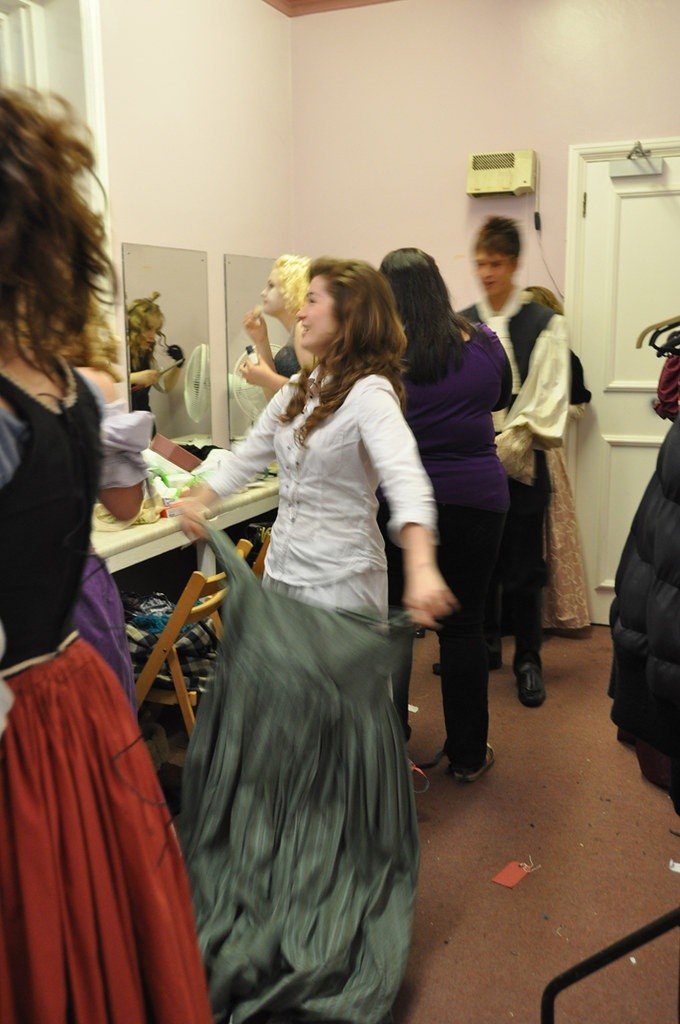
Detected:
[454, 742, 495, 782]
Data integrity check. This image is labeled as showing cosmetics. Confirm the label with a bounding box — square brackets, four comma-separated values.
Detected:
[244, 345, 258, 366]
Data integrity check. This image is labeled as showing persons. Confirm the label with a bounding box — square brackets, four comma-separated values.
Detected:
[126, 293, 186, 438]
[181, 213, 679, 828]
[0, 84, 219, 1024]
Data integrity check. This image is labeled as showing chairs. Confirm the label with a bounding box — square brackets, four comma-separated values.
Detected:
[131, 539, 254, 737]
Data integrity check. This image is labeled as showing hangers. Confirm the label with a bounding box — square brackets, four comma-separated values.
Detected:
[636, 314, 680, 357]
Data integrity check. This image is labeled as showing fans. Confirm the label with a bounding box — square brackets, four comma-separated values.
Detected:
[233, 344, 280, 421]
[183, 344, 210, 423]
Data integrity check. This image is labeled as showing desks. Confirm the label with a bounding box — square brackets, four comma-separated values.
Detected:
[92, 472, 280, 601]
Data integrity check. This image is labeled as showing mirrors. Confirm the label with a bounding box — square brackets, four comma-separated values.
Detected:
[122, 242, 212, 444]
[224, 253, 289, 440]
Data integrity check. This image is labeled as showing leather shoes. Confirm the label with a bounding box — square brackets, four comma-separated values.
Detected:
[433, 651, 502, 676]
[513, 662, 545, 709]
